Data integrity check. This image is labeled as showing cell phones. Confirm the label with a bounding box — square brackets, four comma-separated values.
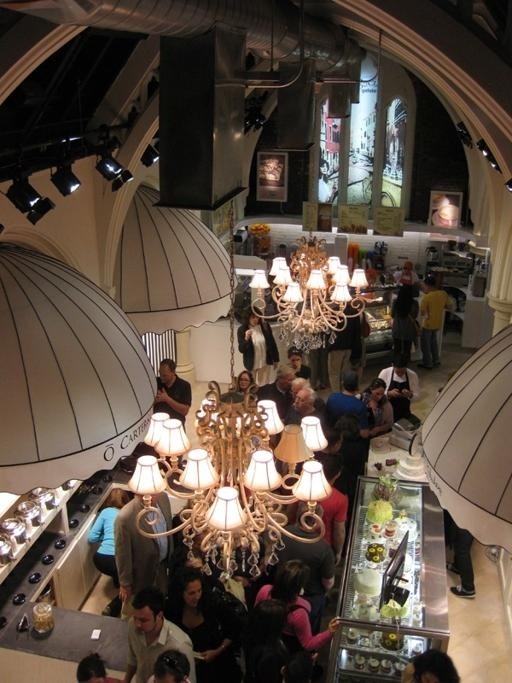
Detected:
[90, 629, 101, 640]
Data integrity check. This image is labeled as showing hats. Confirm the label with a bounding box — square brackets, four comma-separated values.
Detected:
[287, 346, 302, 358]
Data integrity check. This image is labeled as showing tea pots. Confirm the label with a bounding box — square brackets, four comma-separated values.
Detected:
[0, 487, 60, 566]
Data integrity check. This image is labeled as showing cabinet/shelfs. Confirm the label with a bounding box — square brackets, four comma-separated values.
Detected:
[353, 285, 420, 375]
[331, 474, 451, 682]
[0, 459, 133, 612]
[420, 235, 494, 349]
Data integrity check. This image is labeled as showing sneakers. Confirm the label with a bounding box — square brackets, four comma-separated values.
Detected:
[450, 585, 475, 598]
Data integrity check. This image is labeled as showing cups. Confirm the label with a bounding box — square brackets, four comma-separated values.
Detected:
[383, 520, 398, 538]
[346, 241, 373, 276]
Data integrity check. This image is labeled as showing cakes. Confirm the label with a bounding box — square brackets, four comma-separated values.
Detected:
[346, 474, 423, 677]
[394, 434, 428, 482]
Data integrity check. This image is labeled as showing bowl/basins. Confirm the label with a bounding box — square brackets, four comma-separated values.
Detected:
[79, 504, 90, 513]
[41, 553, 54, 565]
[54, 539, 66, 548]
[103, 474, 112, 483]
[28, 572, 41, 583]
[93, 486, 103, 494]
[69, 518, 78, 527]
[12, 593, 26, 604]
[0, 615, 8, 629]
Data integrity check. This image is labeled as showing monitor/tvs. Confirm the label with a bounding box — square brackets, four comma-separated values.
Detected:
[380, 530, 410, 610]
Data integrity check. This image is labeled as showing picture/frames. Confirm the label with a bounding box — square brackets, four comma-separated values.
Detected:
[428, 189, 462, 229]
[256, 150, 288, 201]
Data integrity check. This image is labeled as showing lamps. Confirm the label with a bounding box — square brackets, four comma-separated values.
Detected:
[246, 145, 375, 353]
[111, 184, 237, 336]
[4, 136, 160, 225]
[127, 198, 335, 578]
[1, 240, 160, 495]
[415, 322, 512, 556]
[453, 117, 512, 195]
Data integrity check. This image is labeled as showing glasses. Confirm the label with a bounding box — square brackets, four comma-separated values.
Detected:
[158, 654, 179, 671]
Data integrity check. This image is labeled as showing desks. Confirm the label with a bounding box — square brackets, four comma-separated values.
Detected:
[0, 596, 149, 683]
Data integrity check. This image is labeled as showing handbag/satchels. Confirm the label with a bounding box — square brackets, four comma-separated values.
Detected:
[361, 311, 370, 337]
[415, 325, 422, 335]
